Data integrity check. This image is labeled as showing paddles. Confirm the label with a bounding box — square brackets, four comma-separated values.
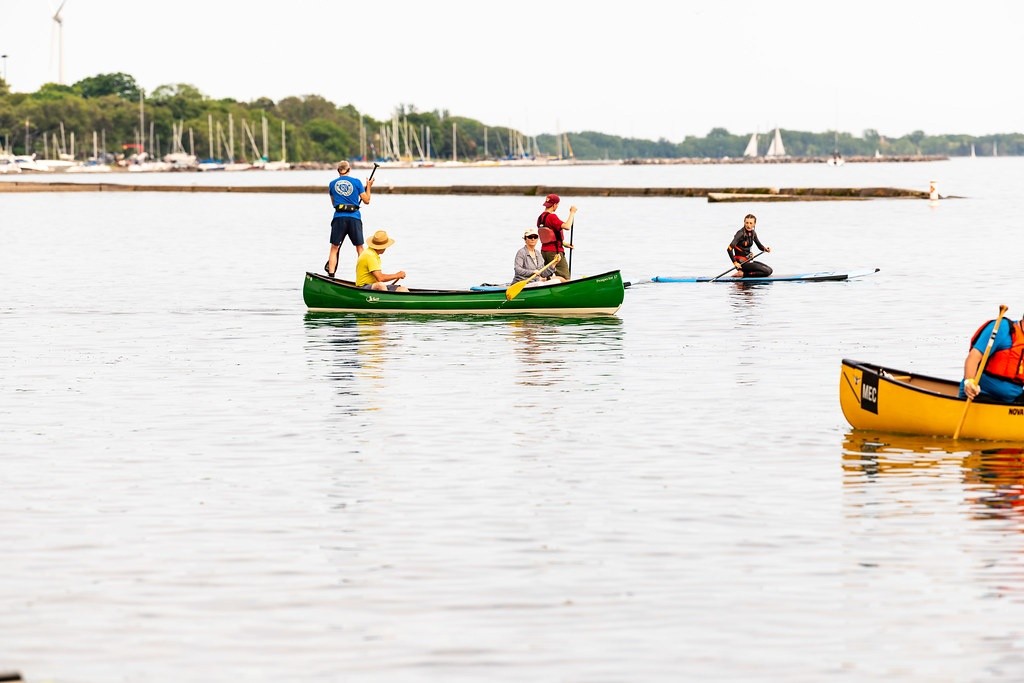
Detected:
[505, 254, 559, 301]
[569, 213, 574, 279]
[709, 248, 769, 283]
[952, 304, 1008, 440]
[392, 278, 401, 285]
[324, 162, 380, 274]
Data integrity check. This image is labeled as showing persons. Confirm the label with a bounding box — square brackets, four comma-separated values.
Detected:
[328, 161, 375, 278]
[537, 194, 577, 280]
[511, 228, 561, 286]
[727, 214, 773, 278]
[356, 230, 410, 292]
[959, 314, 1024, 403]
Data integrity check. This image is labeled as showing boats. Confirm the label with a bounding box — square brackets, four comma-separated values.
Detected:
[840, 356, 1024, 444]
[304, 270, 625, 316]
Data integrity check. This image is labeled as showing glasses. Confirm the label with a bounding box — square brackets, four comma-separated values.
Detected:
[527, 235, 538, 239]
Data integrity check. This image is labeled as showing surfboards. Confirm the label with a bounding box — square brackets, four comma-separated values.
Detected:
[651, 272, 850, 282]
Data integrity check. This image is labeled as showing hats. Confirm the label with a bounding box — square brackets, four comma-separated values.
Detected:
[522, 230, 539, 240]
[366, 230, 395, 250]
[543, 194, 560, 208]
[337, 161, 349, 173]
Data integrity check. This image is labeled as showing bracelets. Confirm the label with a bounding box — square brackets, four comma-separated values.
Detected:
[963, 379, 974, 385]
[563, 242, 567, 247]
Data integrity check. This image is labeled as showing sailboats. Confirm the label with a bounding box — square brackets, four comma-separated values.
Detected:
[0, 86, 621, 174]
[736, 124, 792, 162]
[828, 130, 846, 166]
